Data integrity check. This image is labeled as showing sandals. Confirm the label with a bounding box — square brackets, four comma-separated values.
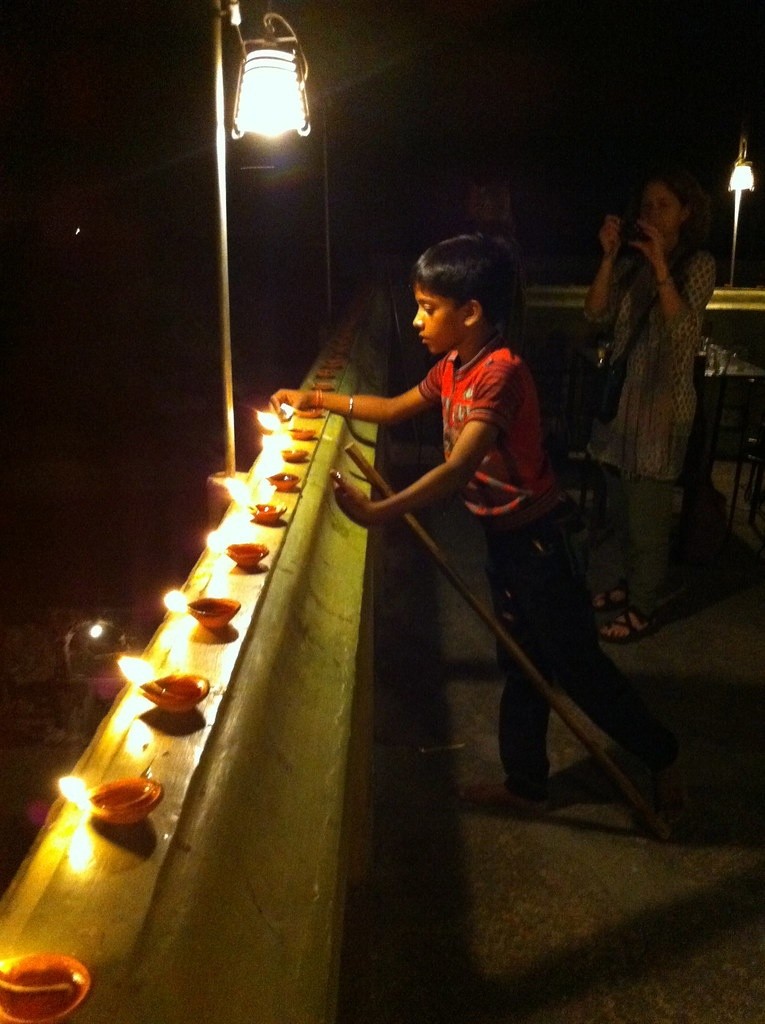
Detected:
[589, 582, 628, 611]
[599, 606, 661, 643]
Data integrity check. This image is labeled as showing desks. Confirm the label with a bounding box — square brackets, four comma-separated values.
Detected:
[562, 335, 765, 560]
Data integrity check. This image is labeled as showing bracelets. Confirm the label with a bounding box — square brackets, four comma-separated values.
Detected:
[315, 389, 323, 408]
[656, 270, 671, 286]
[348, 393, 354, 416]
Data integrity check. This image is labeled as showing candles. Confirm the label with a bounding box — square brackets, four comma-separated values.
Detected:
[163, 588, 242, 631]
[281, 403, 325, 419]
[255, 458, 302, 491]
[0, 953, 92, 1024]
[225, 479, 287, 522]
[117, 656, 212, 715]
[207, 531, 269, 569]
[256, 410, 318, 440]
[58, 772, 164, 826]
[264, 434, 309, 462]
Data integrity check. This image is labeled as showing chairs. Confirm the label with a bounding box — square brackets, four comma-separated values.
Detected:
[537, 383, 607, 542]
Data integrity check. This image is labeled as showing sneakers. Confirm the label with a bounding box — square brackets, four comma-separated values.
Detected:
[458, 783, 550, 824]
[652, 745, 691, 820]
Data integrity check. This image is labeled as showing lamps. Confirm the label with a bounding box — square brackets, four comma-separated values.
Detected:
[727, 137, 756, 193]
[231, 15, 312, 139]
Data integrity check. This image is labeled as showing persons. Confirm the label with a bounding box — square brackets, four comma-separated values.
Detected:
[268, 232, 689, 826]
[584, 173, 717, 645]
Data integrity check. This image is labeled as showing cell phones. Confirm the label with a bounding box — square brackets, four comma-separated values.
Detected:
[617, 221, 645, 241]
